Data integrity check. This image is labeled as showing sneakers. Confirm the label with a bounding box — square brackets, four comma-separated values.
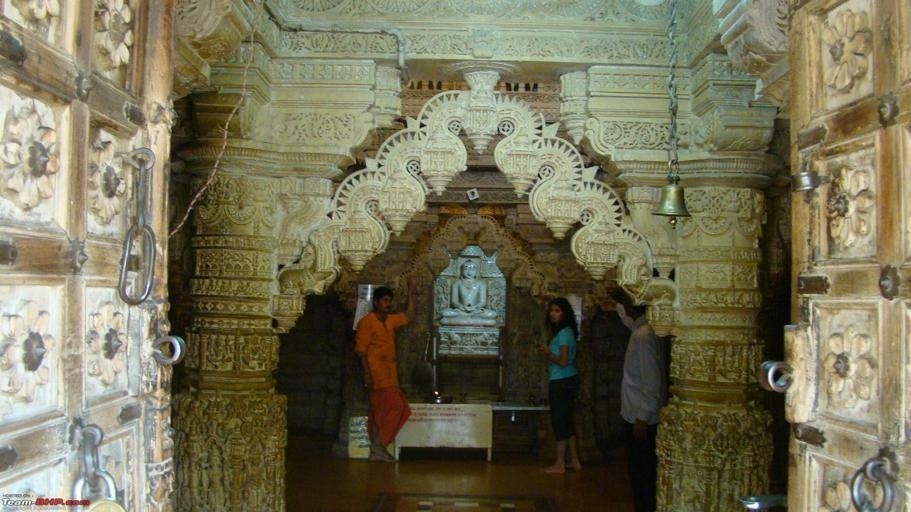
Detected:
[370, 439, 393, 461]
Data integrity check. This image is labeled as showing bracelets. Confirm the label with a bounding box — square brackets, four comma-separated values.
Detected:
[545, 350, 553, 359]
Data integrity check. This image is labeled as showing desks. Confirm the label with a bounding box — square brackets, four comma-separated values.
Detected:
[395, 402, 550, 462]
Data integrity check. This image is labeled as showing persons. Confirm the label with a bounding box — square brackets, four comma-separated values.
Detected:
[538, 297, 586, 474]
[354, 274, 418, 463]
[600, 287, 672, 510]
[439, 258, 499, 327]
